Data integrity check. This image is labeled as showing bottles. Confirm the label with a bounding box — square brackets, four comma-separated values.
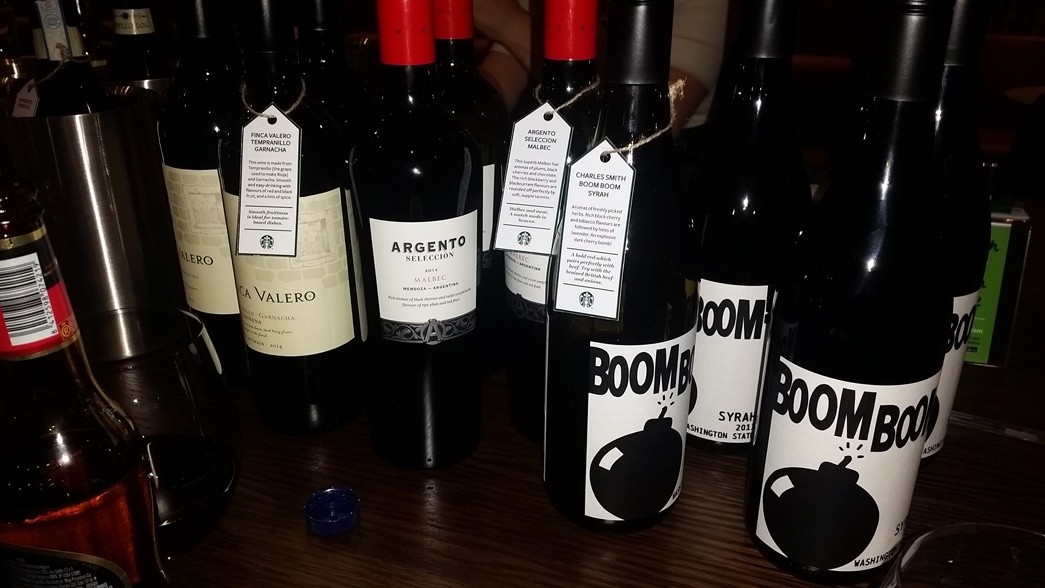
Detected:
[1, 2, 1041, 588]
